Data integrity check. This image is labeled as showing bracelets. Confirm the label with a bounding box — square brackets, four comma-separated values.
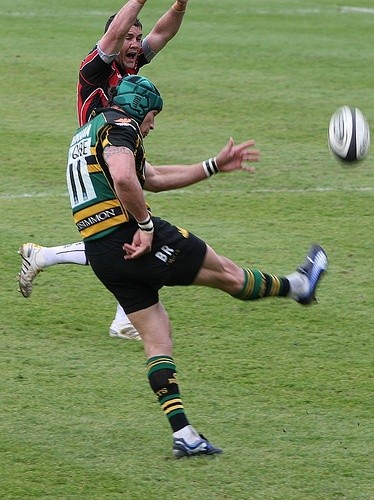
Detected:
[137, 214, 154, 232]
[203, 158, 220, 178]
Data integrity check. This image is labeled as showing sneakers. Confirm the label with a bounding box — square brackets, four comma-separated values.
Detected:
[172, 430, 222, 461]
[294, 244, 327, 306]
[108, 319, 142, 341]
[18, 243, 43, 299]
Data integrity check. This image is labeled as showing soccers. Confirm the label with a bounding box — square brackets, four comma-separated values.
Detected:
[327, 103, 371, 166]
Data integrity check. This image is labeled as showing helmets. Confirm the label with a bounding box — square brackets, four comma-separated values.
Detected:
[110, 74, 164, 127]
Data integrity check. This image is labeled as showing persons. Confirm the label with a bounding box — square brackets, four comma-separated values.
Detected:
[18, 0, 187, 341]
[67, 74, 330, 458]
